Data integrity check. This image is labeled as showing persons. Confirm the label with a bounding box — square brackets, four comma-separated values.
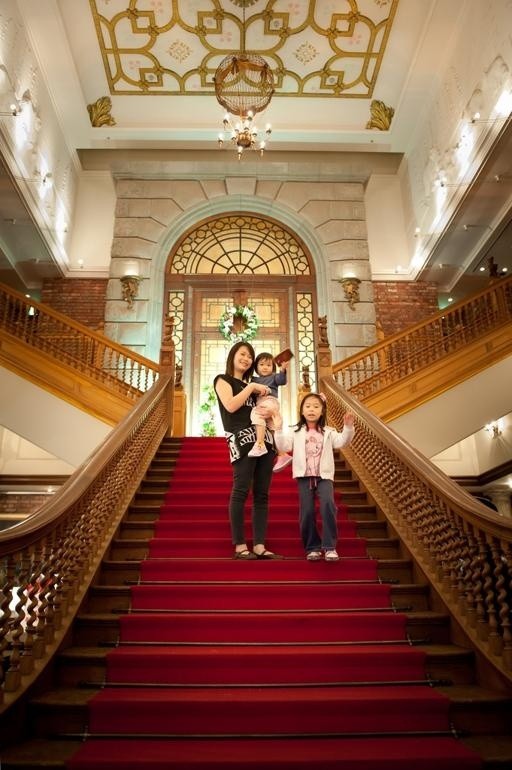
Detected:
[211, 342, 284, 561]
[271, 391, 357, 563]
[247, 352, 294, 473]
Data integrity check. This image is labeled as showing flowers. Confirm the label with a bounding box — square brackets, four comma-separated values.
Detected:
[218, 303, 258, 344]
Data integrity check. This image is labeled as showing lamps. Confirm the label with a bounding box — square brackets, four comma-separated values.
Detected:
[339, 264, 360, 308]
[119, 261, 142, 308]
[213, 51, 275, 160]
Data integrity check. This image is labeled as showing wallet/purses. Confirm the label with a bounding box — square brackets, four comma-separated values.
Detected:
[274, 348, 294, 368]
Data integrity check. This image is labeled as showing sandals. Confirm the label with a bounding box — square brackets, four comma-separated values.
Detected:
[235, 550, 284, 560]
[307, 551, 321, 560]
[325, 552, 339, 561]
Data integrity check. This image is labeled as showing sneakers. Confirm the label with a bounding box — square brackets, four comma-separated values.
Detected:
[272, 452, 293, 473]
[248, 442, 268, 457]
